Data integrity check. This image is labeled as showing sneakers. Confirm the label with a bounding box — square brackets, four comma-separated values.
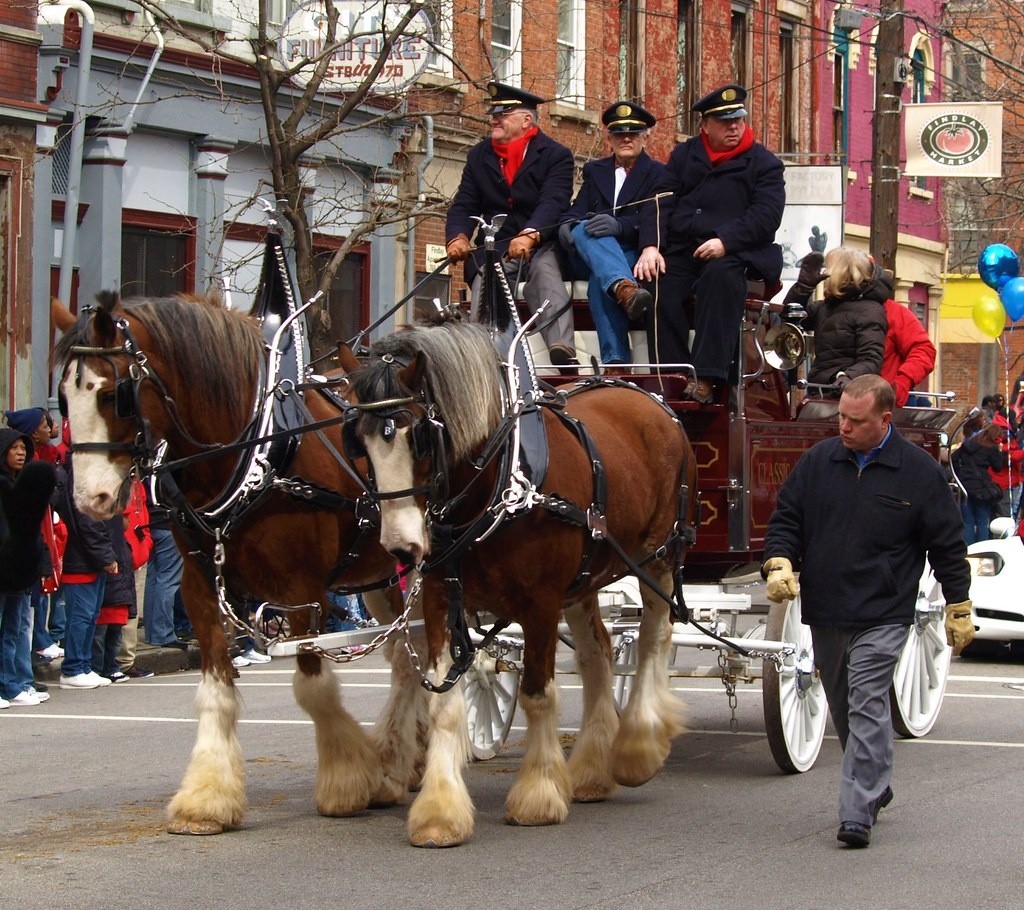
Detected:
[0, 633, 271, 709]
[604, 367, 632, 384]
[683, 378, 714, 403]
[615, 280, 652, 320]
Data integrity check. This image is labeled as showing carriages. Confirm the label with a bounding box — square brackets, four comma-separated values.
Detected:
[49, 276, 958, 851]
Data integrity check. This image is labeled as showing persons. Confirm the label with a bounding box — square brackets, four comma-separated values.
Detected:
[762, 374, 978, 846]
[781, 246, 890, 418]
[647, 84, 787, 403]
[865, 252, 939, 407]
[445, 83, 579, 377]
[222, 562, 408, 667]
[558, 100, 672, 376]
[0, 408, 199, 709]
[906, 370, 1024, 545]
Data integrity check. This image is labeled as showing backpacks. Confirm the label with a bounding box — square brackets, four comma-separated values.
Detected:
[960, 444, 982, 503]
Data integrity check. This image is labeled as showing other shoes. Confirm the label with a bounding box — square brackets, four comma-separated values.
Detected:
[549, 344, 579, 374]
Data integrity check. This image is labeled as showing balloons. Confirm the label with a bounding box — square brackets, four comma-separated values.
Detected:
[978, 244, 1021, 296]
[972, 296, 1007, 338]
[1002, 277, 1024, 322]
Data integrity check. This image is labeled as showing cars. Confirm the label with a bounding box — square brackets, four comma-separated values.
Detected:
[966, 517, 1024, 656]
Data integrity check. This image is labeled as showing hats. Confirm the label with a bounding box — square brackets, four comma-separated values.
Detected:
[5, 409, 42, 435]
[485, 81, 545, 115]
[601, 101, 656, 133]
[691, 85, 748, 119]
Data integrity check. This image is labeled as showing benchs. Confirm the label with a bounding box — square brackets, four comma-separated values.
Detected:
[457, 263, 790, 415]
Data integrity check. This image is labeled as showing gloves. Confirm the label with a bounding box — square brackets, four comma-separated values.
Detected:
[830, 376, 851, 395]
[944, 599, 975, 656]
[583, 212, 622, 237]
[797, 251, 830, 290]
[762, 556, 799, 603]
[558, 219, 580, 251]
[890, 374, 912, 407]
[505, 228, 540, 262]
[447, 235, 471, 266]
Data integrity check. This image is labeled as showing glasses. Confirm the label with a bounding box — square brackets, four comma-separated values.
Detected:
[999, 399, 1003, 401]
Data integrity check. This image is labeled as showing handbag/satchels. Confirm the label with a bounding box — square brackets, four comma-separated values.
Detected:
[979, 470, 1003, 502]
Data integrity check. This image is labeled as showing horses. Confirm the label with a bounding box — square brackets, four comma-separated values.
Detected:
[48, 286, 445, 834]
[334, 320, 696, 851]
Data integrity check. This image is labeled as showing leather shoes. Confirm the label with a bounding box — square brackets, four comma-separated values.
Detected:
[836, 821, 871, 844]
[873, 785, 893, 824]
